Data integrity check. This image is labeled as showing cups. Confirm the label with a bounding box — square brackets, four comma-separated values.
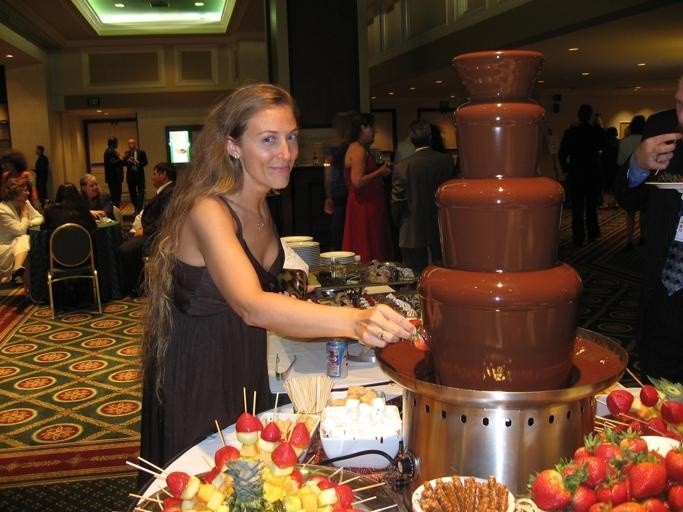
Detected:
[378, 261, 399, 282]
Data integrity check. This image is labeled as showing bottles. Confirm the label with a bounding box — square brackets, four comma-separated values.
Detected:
[329, 256, 343, 278]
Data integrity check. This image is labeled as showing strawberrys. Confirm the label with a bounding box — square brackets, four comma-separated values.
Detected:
[162, 413, 365, 512]
[532, 383, 683, 512]
[411, 332, 429, 351]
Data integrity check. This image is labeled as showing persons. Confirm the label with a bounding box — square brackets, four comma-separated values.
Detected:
[138, 83, 417, 500]
[341, 113, 391, 264]
[558, 77, 683, 385]
[0, 136, 177, 301]
[391, 118, 457, 289]
[322, 133, 352, 252]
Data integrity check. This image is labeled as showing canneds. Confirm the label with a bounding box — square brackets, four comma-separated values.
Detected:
[325, 338, 348, 379]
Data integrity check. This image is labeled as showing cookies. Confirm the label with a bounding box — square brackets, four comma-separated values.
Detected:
[421, 475, 509, 512]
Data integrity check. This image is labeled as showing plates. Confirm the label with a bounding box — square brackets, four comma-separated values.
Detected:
[321, 251, 356, 266]
[645, 182, 683, 190]
[411, 475, 516, 512]
[280, 235, 321, 266]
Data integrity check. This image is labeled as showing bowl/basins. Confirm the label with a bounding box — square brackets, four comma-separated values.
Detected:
[320, 403, 401, 467]
[225, 409, 320, 463]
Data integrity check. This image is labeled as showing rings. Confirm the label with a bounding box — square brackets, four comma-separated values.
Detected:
[378, 328, 383, 341]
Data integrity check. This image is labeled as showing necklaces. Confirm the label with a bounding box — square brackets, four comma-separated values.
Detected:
[257, 220, 264, 232]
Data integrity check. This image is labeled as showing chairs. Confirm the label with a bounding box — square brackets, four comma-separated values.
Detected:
[46, 222, 104, 317]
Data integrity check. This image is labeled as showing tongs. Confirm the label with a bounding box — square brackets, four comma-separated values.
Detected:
[273, 352, 298, 380]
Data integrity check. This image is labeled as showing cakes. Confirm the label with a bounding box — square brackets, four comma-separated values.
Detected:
[317, 260, 422, 320]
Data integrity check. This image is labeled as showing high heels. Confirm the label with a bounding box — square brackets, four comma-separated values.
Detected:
[10, 266, 26, 280]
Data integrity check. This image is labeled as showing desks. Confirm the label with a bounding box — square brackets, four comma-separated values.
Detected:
[135, 382, 681, 512]
[266, 327, 390, 397]
